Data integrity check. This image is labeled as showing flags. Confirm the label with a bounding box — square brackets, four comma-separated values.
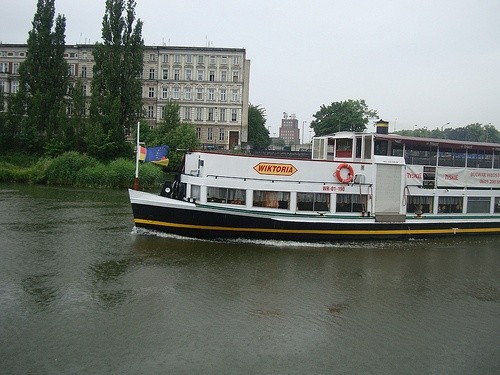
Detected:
[142, 144, 170, 164]
[138, 145, 169, 167]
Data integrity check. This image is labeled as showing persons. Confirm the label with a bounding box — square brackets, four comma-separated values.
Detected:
[438, 204, 446, 213]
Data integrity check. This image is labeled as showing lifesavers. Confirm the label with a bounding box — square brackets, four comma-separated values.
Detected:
[336, 163, 353, 183]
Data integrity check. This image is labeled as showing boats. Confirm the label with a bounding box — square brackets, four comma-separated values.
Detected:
[125, 119, 500, 238]
